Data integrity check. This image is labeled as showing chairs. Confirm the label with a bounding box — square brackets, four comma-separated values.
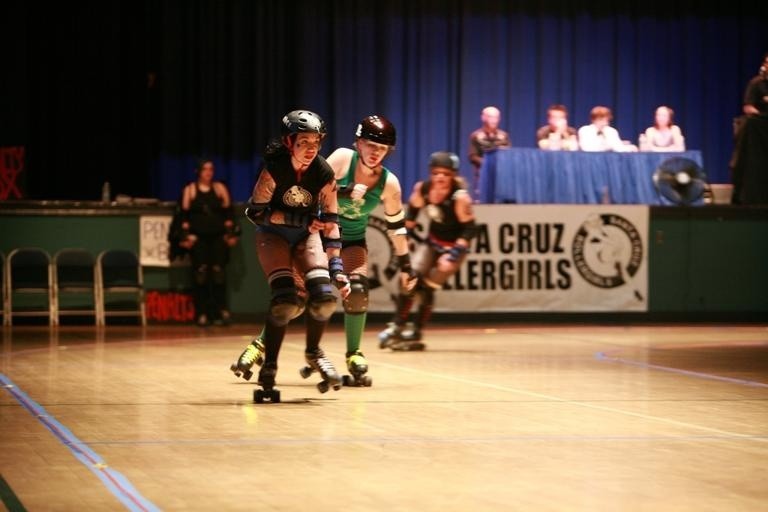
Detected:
[49, 245, 101, 332]
[93, 247, 148, 330]
[1, 243, 53, 333]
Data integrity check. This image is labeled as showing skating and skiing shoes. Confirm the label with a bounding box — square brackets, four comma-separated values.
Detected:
[300, 348, 341, 393]
[230, 336, 265, 381]
[253, 362, 279, 402]
[378, 323, 423, 350]
[342, 351, 371, 386]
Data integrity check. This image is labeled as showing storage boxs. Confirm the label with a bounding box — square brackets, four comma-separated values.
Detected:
[701, 183, 734, 205]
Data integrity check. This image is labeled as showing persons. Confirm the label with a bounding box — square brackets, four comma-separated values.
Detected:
[468, 105, 513, 205]
[537, 104, 580, 152]
[378, 150, 474, 341]
[245, 109, 352, 385]
[576, 106, 630, 153]
[178, 158, 240, 327]
[644, 106, 687, 153]
[238, 114, 418, 373]
[743, 57, 768, 116]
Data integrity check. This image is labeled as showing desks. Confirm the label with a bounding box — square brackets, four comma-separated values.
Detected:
[479, 142, 709, 207]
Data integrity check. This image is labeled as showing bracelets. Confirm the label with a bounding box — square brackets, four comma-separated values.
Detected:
[329, 257, 344, 271]
[450, 243, 466, 257]
[397, 254, 412, 272]
[284, 212, 306, 228]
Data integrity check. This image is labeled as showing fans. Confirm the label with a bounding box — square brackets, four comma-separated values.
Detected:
[648, 156, 711, 205]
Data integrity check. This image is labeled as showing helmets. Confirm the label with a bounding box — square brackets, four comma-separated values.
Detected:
[428, 151, 459, 171]
[354, 114, 394, 147]
[280, 110, 326, 139]
